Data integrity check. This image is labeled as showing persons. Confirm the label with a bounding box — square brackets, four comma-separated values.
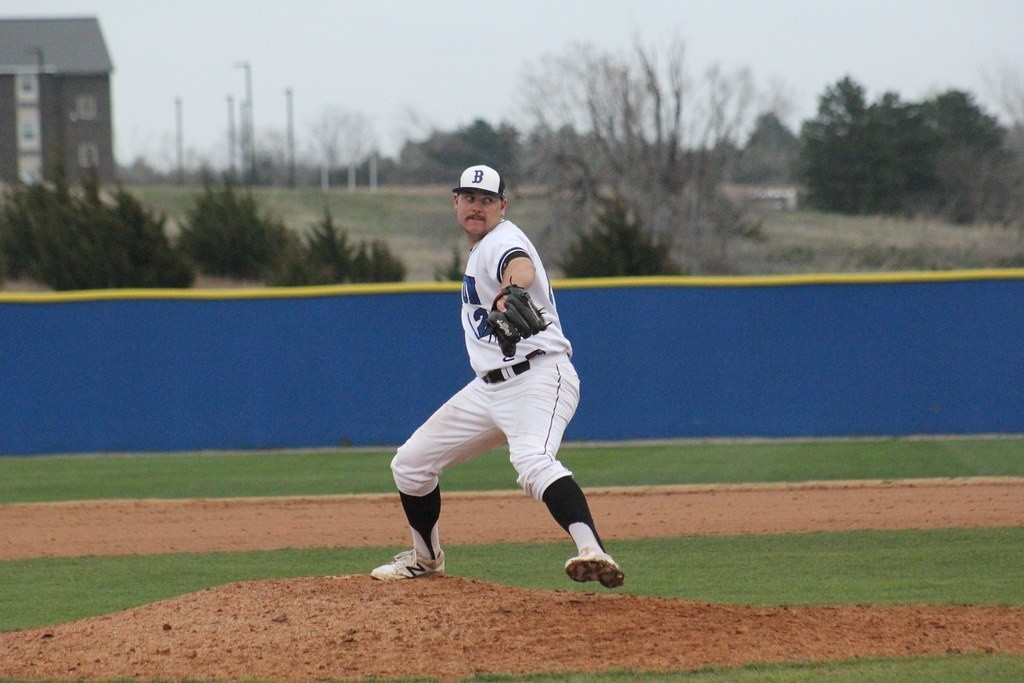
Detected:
[370, 164, 626, 588]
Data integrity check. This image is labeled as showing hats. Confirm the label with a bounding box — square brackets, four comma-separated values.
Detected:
[452, 164, 505, 197]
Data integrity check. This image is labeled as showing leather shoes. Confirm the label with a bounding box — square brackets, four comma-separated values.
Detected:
[483, 361, 531, 384]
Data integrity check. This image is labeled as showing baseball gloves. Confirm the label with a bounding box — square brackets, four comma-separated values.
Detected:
[485, 283, 548, 358]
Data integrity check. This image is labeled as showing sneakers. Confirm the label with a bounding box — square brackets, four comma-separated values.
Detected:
[564, 548, 626, 588]
[370, 546, 445, 581]
